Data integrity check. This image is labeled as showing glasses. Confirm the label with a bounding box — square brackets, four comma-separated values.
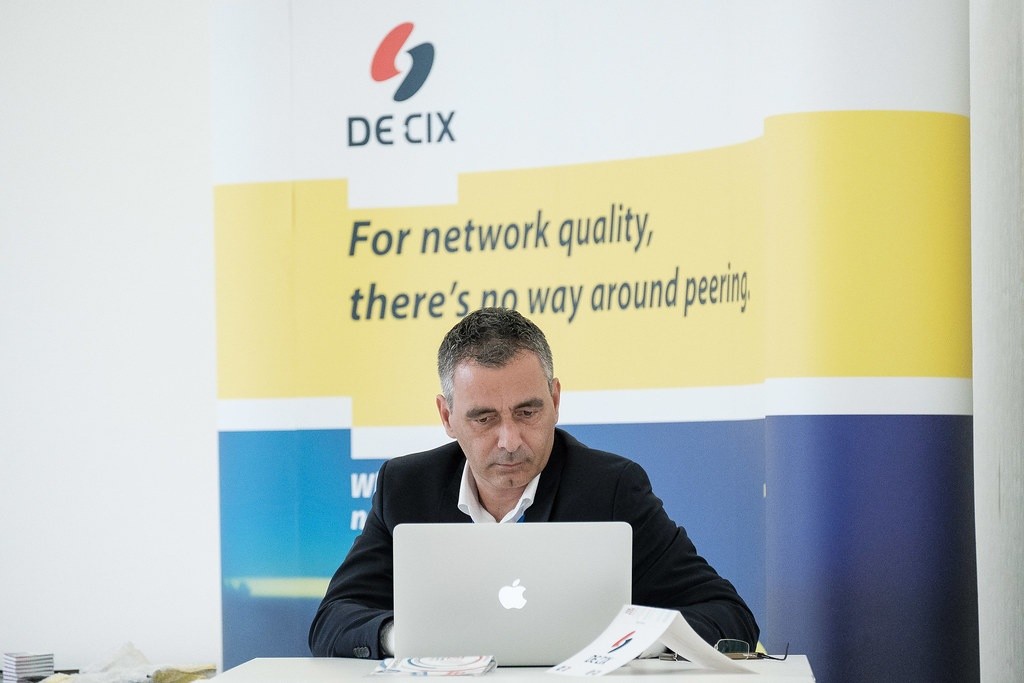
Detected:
[674, 639, 791, 664]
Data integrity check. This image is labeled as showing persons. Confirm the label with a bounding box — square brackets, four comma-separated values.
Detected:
[307, 307, 760, 660]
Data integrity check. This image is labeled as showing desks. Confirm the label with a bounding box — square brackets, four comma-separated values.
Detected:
[208, 655, 816, 683]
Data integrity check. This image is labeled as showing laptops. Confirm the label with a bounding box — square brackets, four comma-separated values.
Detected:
[392, 522, 633, 668]
